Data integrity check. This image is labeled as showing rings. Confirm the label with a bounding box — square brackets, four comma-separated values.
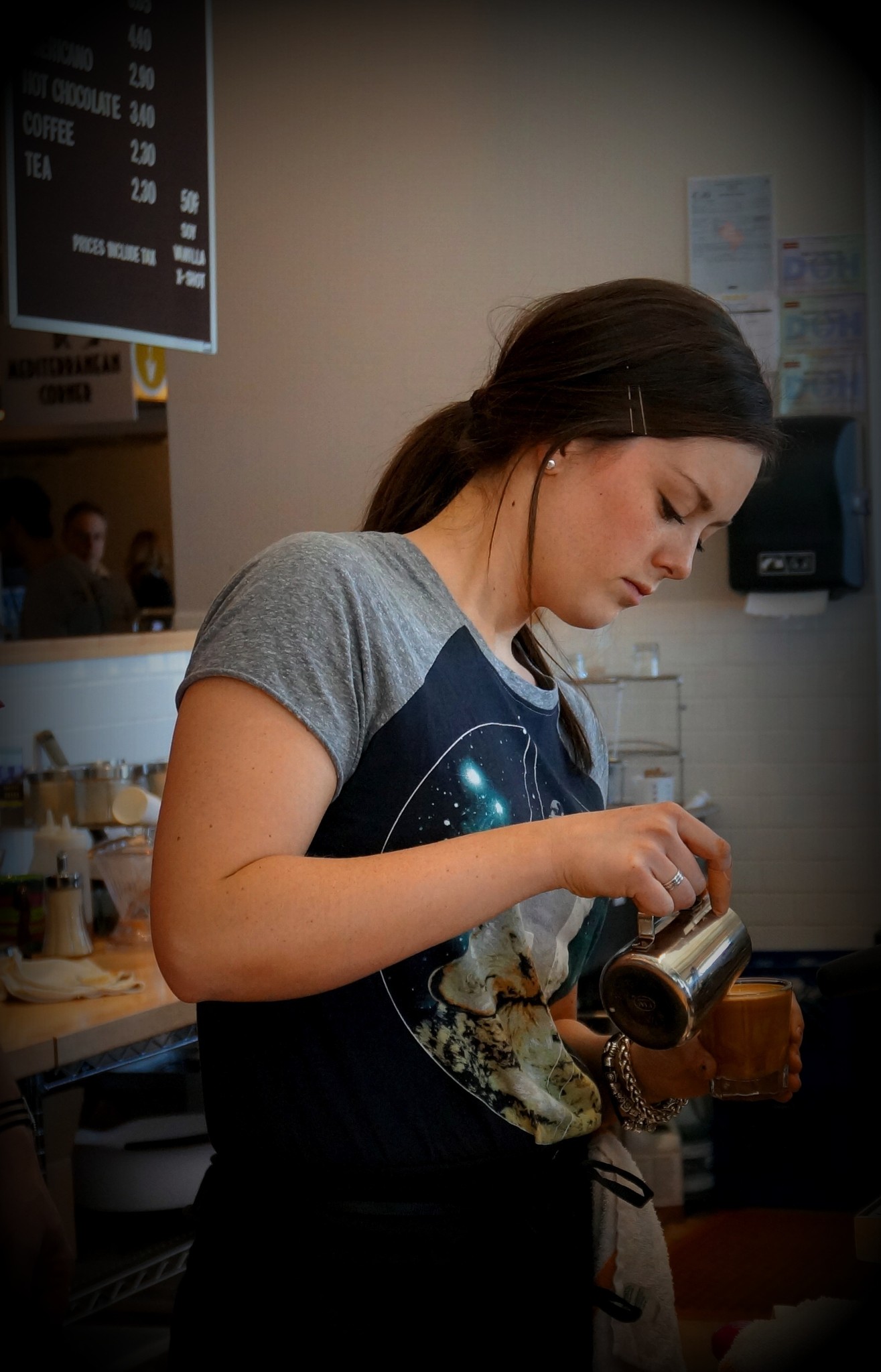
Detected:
[663, 869, 684, 891]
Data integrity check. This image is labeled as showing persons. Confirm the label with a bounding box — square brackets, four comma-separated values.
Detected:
[125, 533, 173, 608]
[151, 278, 805, 1372]
[19, 500, 138, 640]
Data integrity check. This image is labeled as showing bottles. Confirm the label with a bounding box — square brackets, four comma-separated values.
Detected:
[27, 760, 167, 823]
[29, 810, 93, 956]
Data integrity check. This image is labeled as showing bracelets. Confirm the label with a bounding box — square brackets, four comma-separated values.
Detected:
[600, 1032, 689, 1134]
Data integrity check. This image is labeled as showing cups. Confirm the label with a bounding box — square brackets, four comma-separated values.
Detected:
[93, 851, 154, 950]
[112, 787, 162, 825]
[706, 978, 793, 1098]
[0, 874, 45, 954]
[633, 641, 660, 676]
[643, 774, 675, 804]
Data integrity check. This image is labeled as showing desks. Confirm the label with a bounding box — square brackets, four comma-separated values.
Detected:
[0, 927, 199, 1372]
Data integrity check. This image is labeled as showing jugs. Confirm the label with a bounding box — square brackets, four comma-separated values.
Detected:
[599, 887, 752, 1050]
